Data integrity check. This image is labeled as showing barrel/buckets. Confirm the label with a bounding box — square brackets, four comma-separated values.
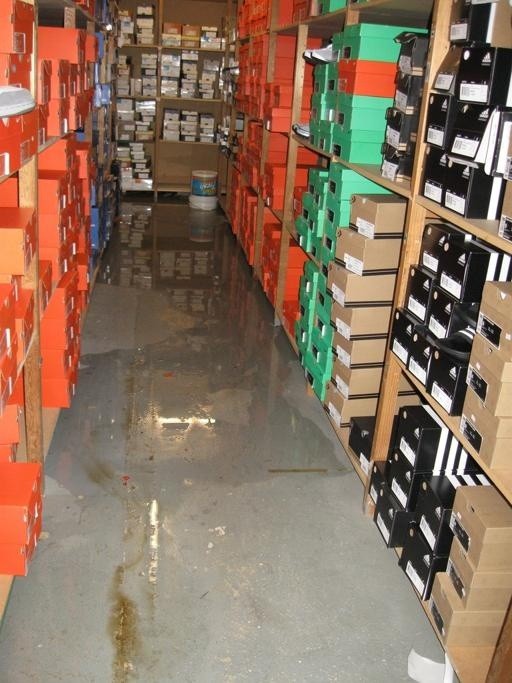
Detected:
[188, 211, 215, 243]
[189, 170, 218, 211]
